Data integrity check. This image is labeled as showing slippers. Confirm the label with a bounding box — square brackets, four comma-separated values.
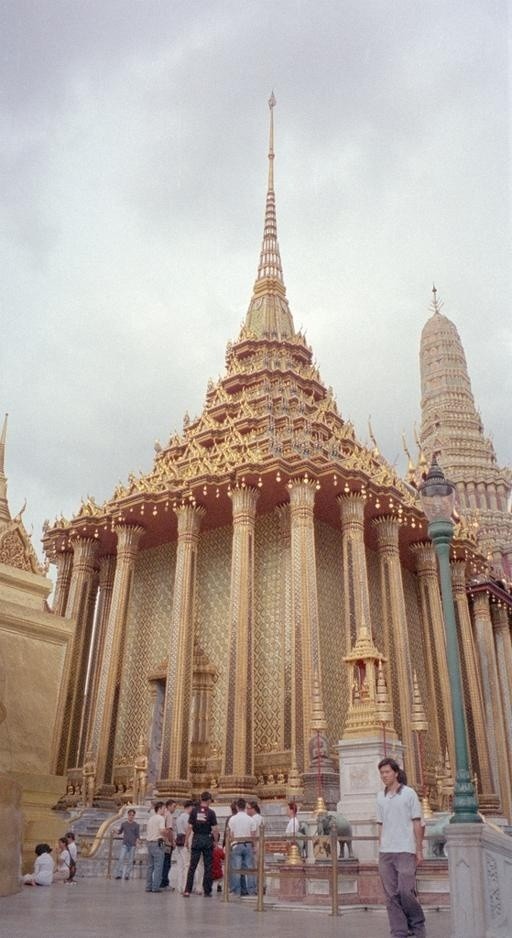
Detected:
[63, 850, 75, 881]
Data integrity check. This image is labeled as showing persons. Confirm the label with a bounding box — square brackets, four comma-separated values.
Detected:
[24, 844, 54, 886]
[144, 792, 264, 897]
[112, 809, 139, 880]
[54, 838, 70, 884]
[65, 832, 77, 880]
[397, 768, 426, 840]
[378, 757, 428, 938]
[285, 802, 299, 833]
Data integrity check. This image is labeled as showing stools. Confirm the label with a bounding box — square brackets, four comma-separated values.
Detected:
[232, 841, 253, 847]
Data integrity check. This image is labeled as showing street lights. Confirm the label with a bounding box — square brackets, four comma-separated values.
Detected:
[159, 838, 172, 854]
[176, 833, 185, 846]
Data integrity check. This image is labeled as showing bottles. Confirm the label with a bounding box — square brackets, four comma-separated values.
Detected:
[201, 792, 214, 802]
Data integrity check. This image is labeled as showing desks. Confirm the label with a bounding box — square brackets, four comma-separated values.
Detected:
[419, 454, 484, 822]
